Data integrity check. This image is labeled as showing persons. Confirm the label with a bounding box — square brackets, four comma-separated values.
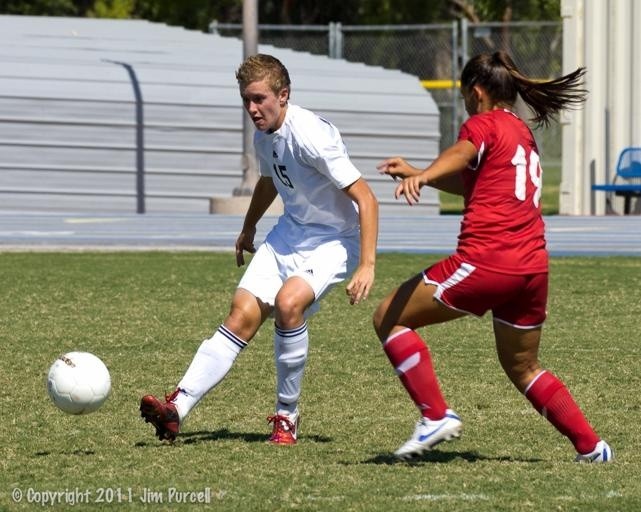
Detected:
[140, 54, 379, 446]
[373, 50, 615, 464]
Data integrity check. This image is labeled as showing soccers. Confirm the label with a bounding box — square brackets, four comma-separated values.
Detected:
[47, 351, 112, 416]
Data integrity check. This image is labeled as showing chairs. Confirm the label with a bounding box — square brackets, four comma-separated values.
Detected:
[591, 147, 640, 214]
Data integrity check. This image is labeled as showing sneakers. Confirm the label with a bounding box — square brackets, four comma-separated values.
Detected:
[139, 394, 180, 443]
[266, 408, 301, 445]
[574, 439, 615, 463]
[393, 407, 464, 461]
